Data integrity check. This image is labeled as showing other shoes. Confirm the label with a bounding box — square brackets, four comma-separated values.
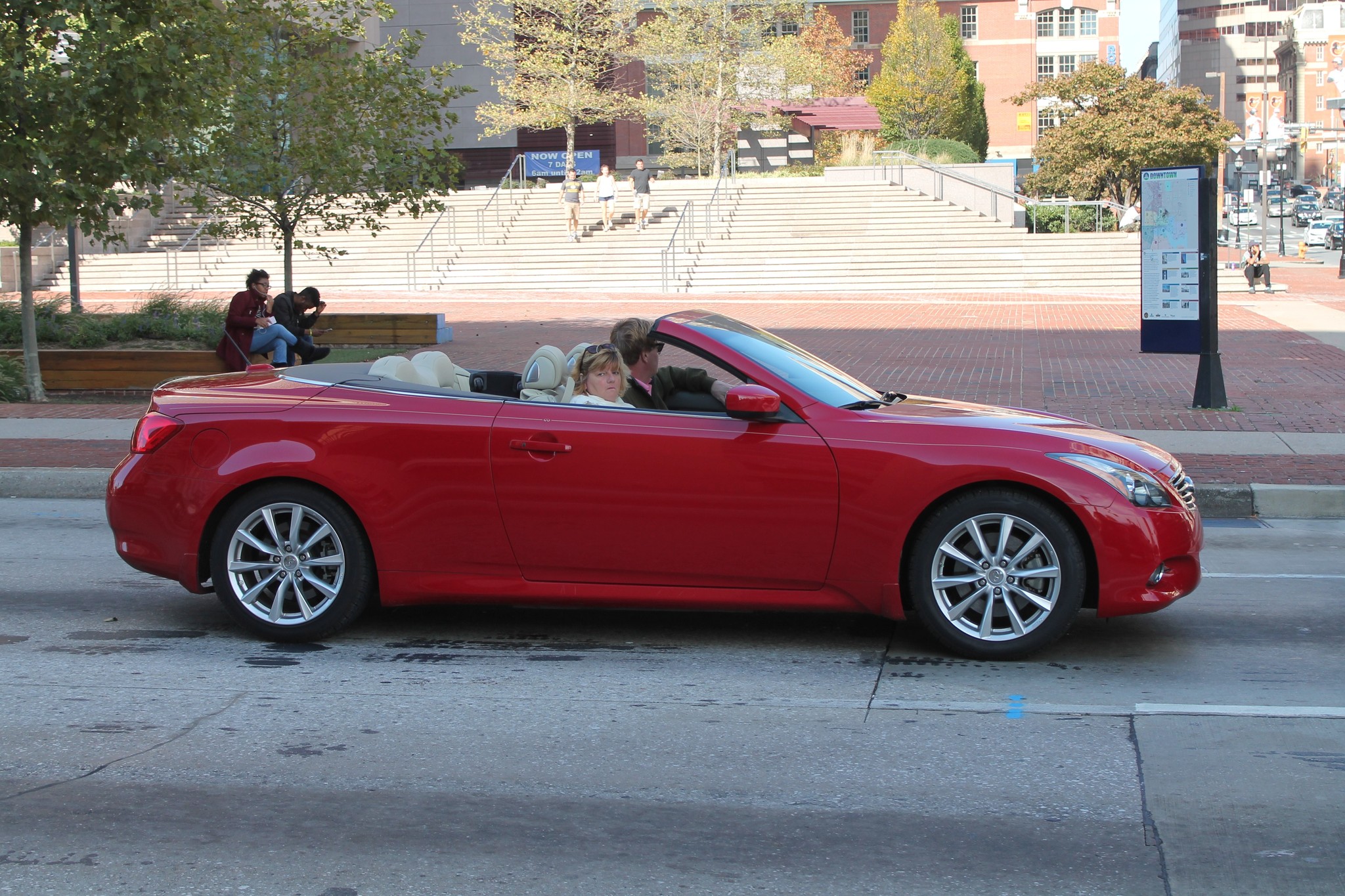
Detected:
[567, 231, 578, 242]
[603, 220, 613, 232]
[636, 220, 647, 231]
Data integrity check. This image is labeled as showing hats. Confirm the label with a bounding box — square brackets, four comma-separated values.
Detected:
[1136, 201, 1140, 208]
[1248, 240, 1259, 248]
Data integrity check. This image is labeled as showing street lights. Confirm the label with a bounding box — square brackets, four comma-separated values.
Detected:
[1234, 154, 1245, 249]
[1276, 141, 1287, 256]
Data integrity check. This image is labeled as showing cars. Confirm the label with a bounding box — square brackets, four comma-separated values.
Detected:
[1220, 185, 1345, 249]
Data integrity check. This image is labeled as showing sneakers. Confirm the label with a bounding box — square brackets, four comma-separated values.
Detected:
[1264, 289, 1274, 294]
[1248, 289, 1256, 294]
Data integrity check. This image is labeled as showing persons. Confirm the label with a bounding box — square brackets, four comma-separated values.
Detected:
[569, 342, 635, 408]
[1243, 240, 1274, 293]
[556, 169, 585, 242]
[629, 159, 654, 232]
[216, 269, 331, 373]
[270, 287, 326, 365]
[594, 164, 617, 232]
[608, 317, 736, 410]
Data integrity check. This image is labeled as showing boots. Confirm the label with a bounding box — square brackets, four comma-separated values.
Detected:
[271, 335, 330, 369]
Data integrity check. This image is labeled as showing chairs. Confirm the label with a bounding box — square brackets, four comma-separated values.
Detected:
[368, 355, 429, 385]
[565, 342, 593, 373]
[520, 345, 570, 403]
[411, 351, 470, 391]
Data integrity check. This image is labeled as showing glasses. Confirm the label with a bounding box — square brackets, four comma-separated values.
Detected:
[655, 342, 665, 354]
[254, 282, 271, 290]
[579, 343, 616, 371]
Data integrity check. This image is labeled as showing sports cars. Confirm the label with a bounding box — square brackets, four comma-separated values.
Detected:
[102, 309, 1208, 658]
